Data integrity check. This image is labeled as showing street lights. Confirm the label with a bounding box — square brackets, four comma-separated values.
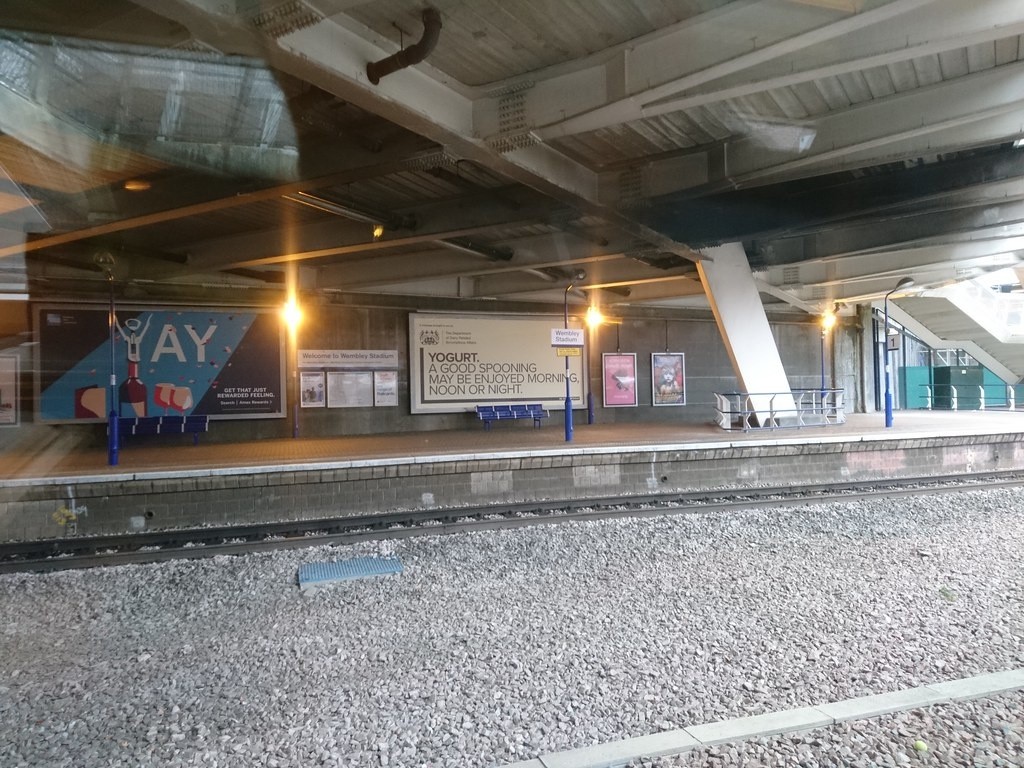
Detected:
[94, 249, 120, 466]
[282, 299, 302, 440]
[563, 269, 587, 441]
[884, 277, 915, 428]
[585, 307, 602, 426]
[820, 313, 836, 415]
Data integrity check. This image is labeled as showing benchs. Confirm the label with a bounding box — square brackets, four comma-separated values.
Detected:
[475, 404, 551, 431]
[107, 415, 211, 448]
